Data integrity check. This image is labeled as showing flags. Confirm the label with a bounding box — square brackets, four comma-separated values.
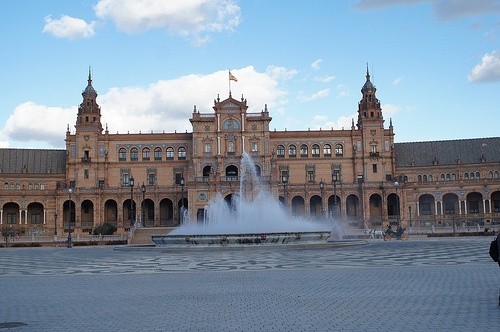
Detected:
[230, 72, 237, 81]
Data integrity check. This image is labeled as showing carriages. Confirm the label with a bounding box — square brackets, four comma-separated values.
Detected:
[364, 227, 409, 242]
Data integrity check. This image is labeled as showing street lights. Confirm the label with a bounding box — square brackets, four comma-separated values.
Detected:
[179, 177, 185, 208]
[141, 185, 146, 226]
[54, 214, 57, 235]
[394, 182, 400, 224]
[129, 177, 135, 225]
[282, 175, 288, 204]
[67, 189, 73, 248]
[319, 181, 325, 214]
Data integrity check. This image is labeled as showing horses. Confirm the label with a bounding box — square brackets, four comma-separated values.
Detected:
[364, 228, 385, 240]
